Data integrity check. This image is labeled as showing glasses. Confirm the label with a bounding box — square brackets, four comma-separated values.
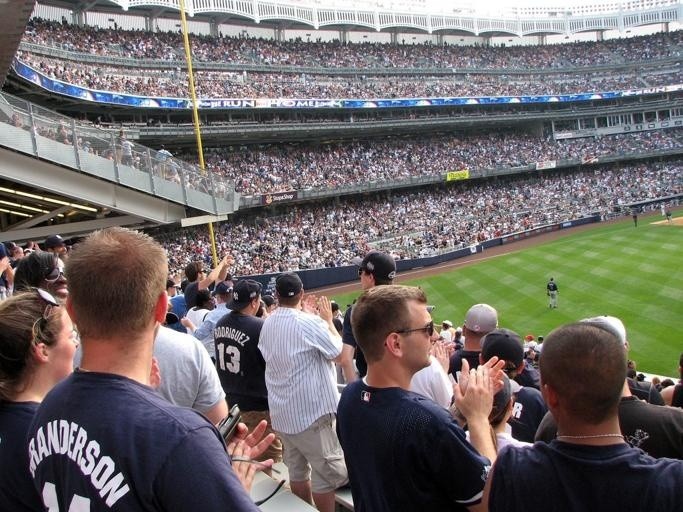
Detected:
[30, 287, 60, 342]
[230, 459, 285, 507]
[42, 267, 66, 282]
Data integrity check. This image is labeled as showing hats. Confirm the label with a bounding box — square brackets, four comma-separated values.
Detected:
[45, 234, 66, 247]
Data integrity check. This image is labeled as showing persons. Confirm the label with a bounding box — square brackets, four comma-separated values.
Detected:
[1, 13, 683, 511]
